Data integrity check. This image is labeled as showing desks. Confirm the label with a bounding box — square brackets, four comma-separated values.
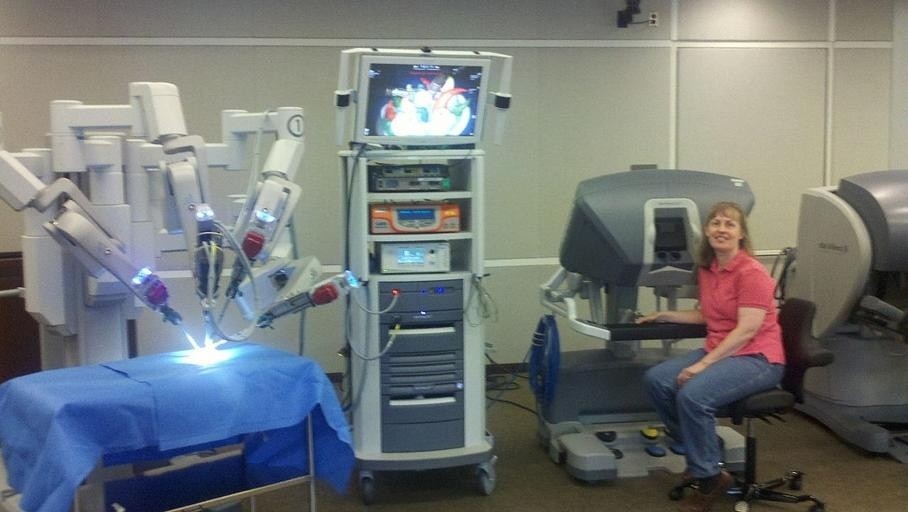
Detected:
[1, 342, 326, 512]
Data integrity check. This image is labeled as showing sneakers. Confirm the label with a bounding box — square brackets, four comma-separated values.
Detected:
[679, 463, 734, 512]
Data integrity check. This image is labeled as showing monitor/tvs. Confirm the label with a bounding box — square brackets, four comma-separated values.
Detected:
[353, 54, 491, 146]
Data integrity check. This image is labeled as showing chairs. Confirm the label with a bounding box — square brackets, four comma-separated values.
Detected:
[715, 296, 832, 512]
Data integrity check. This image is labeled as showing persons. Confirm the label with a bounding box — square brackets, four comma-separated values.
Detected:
[633, 201, 785, 510]
